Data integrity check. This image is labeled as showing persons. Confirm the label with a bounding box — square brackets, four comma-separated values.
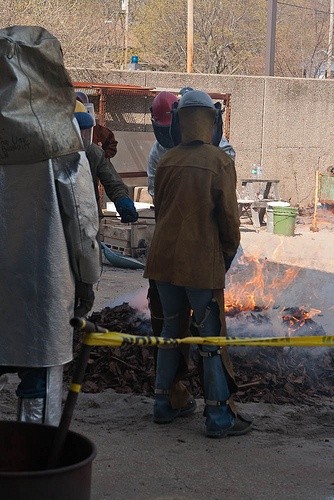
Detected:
[0, 25, 139, 428]
[143, 89, 254, 438]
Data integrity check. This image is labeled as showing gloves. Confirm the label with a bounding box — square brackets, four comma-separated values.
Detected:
[73, 284, 95, 319]
[117, 197, 138, 223]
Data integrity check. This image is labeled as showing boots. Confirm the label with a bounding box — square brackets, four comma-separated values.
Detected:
[16, 398, 46, 424]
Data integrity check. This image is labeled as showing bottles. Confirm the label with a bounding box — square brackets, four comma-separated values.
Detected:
[251, 163, 257, 178]
[256, 163, 263, 179]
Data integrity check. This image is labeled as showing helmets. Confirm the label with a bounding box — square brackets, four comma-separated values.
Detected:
[180, 87, 193, 96]
[73, 100, 86, 112]
[150, 92, 179, 127]
[176, 90, 214, 108]
[75, 91, 88, 103]
[75, 113, 94, 129]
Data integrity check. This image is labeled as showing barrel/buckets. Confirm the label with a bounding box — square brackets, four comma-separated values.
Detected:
[272, 206, 298, 237]
[0, 419, 96, 500]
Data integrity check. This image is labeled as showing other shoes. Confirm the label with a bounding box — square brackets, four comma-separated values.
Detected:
[205, 417, 253, 438]
[153, 401, 197, 424]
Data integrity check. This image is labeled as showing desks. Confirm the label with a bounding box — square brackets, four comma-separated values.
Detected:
[237, 199, 283, 233]
